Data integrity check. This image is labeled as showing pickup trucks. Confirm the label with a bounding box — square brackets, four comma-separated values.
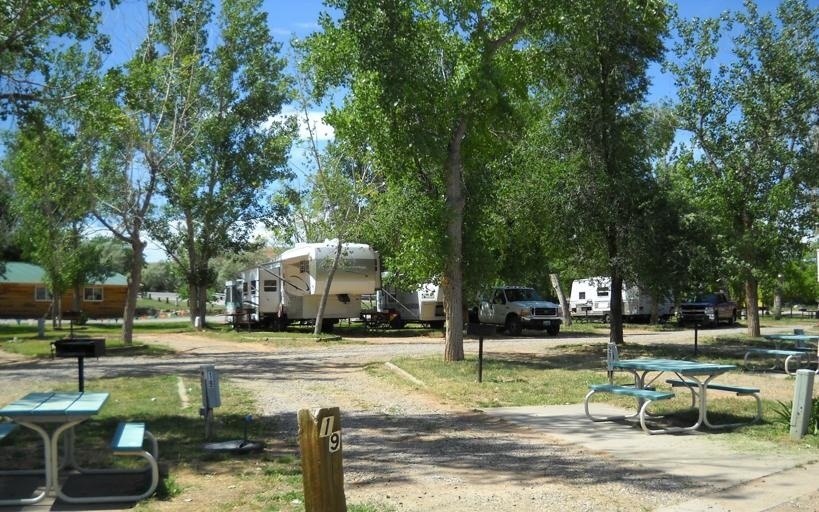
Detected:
[676, 291, 738, 328]
[468, 286, 561, 335]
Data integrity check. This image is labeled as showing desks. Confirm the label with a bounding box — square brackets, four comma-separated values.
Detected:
[762, 333, 819, 376]
[0, 390, 111, 505]
[605, 355, 737, 431]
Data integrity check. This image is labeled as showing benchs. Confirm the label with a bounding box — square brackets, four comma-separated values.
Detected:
[584, 377, 773, 436]
[1, 418, 161, 506]
[743, 344, 819, 375]
